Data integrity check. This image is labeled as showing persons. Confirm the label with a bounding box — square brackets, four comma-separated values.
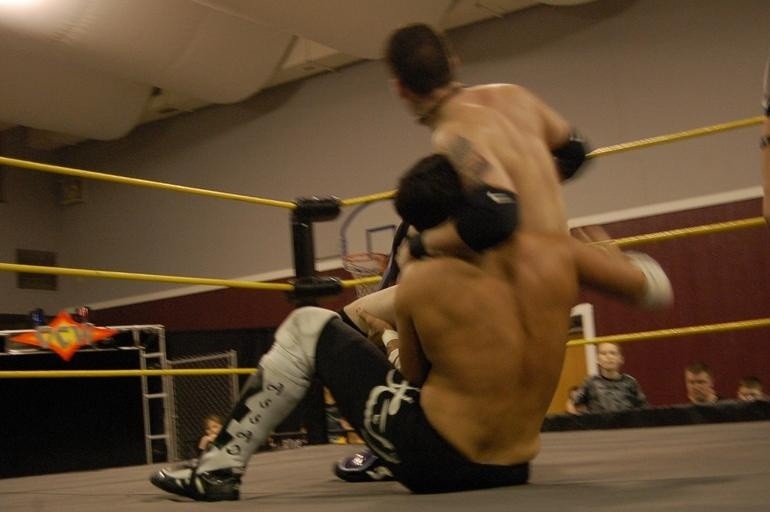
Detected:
[565, 341, 649, 416]
[298, 383, 360, 445]
[334, 24, 587, 482]
[739, 378, 766, 400]
[152, 154, 674, 501]
[190, 415, 224, 458]
[684, 363, 724, 406]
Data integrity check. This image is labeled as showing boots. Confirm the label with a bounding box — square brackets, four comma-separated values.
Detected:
[150, 353, 310, 501]
[334, 447, 394, 483]
